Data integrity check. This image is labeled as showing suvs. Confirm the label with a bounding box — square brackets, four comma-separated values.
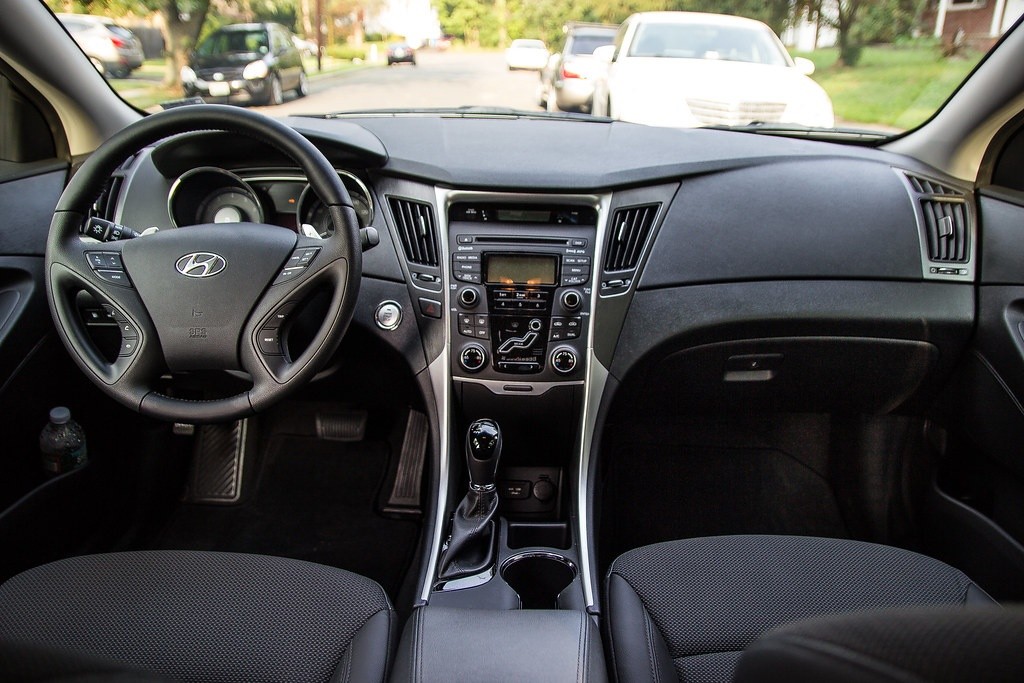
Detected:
[536, 22, 620, 113]
[54, 12, 145, 77]
[180, 21, 309, 106]
[386, 41, 415, 66]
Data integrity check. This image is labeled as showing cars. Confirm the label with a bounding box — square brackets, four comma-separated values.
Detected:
[292, 34, 319, 58]
[505, 39, 550, 70]
[591, 10, 835, 131]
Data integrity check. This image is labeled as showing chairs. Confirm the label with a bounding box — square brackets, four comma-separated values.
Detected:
[0, 551, 392, 682]
[599, 534, 1023, 682]
